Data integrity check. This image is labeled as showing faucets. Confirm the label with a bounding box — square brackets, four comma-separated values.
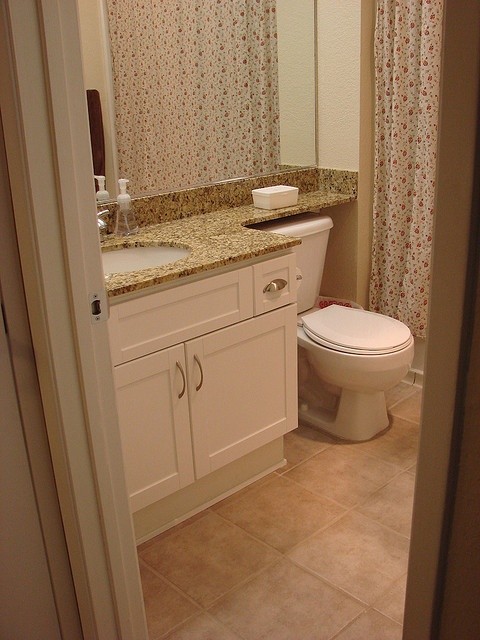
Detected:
[96, 209, 110, 243]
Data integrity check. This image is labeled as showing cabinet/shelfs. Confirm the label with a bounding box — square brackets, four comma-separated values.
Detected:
[105, 249, 299, 545]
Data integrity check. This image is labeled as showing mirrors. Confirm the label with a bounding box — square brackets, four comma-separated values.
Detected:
[77, 0, 320, 204]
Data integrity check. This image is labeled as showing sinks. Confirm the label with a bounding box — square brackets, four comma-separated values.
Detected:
[99, 240, 196, 278]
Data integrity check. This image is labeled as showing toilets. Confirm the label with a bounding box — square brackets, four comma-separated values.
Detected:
[255, 214, 415, 441]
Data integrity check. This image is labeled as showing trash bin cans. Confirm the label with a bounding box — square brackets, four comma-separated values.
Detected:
[314, 296, 364, 310]
